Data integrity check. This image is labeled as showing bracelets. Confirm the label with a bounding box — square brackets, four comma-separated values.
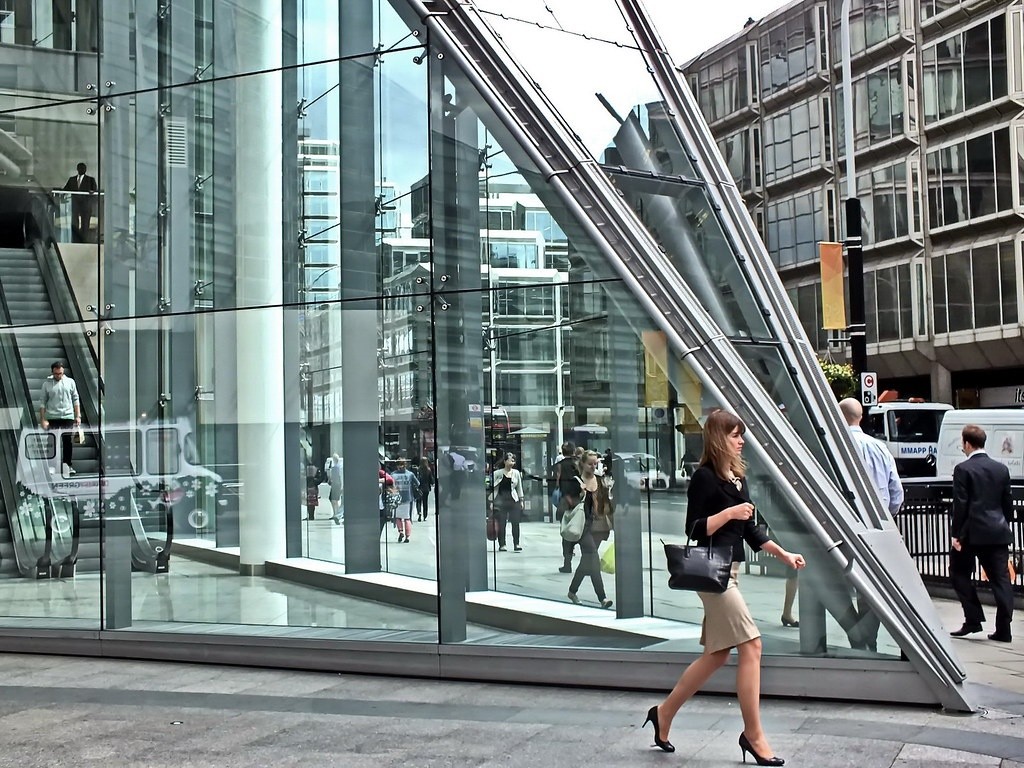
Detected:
[75, 416, 81, 418]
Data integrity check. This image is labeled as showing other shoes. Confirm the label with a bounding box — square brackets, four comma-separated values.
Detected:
[514, 548, 522, 551]
[329, 516, 344, 525]
[499, 547, 507, 551]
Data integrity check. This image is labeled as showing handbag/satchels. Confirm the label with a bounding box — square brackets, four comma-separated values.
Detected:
[660, 518, 734, 594]
[73, 425, 85, 444]
[411, 481, 423, 499]
[487, 510, 499, 541]
[600, 544, 615, 574]
[551, 463, 561, 507]
[307, 495, 318, 506]
[382, 484, 402, 509]
[561, 502, 585, 542]
[590, 515, 612, 532]
[682, 471, 685, 477]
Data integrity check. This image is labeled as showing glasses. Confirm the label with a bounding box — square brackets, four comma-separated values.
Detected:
[53, 372, 62, 375]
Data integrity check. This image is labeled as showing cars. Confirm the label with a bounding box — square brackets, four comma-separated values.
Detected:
[617, 452, 670, 492]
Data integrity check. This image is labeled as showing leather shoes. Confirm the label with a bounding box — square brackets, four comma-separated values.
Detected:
[601, 601, 613, 610]
[950, 623, 983, 636]
[988, 631, 1012, 642]
[568, 591, 582, 605]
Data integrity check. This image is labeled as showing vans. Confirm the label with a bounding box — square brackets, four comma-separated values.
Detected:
[937, 408, 1024, 485]
[19, 417, 223, 533]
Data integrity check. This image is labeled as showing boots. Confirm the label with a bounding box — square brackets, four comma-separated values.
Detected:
[418, 513, 425, 522]
[559, 561, 571, 573]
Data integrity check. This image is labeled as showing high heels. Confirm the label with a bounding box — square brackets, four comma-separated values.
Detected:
[781, 616, 800, 627]
[642, 706, 676, 752]
[739, 731, 784, 766]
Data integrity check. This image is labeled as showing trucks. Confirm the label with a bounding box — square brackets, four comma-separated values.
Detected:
[869, 403, 955, 514]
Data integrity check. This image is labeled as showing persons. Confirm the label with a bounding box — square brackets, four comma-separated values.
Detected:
[307, 447, 612, 572]
[680, 449, 698, 477]
[839, 398, 905, 651]
[52, 163, 97, 245]
[781, 565, 798, 628]
[40, 361, 82, 477]
[642, 410, 805, 766]
[564, 451, 613, 609]
[950, 426, 1013, 642]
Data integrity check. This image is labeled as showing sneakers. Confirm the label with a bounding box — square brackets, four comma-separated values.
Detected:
[398, 534, 404, 542]
[69, 467, 77, 475]
[404, 539, 410, 543]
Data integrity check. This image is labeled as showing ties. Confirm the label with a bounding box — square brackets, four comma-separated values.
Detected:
[77, 175, 81, 189]
